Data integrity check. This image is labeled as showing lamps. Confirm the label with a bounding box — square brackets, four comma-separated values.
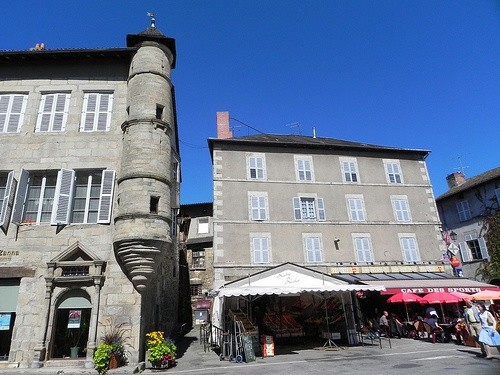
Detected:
[441, 227, 457, 242]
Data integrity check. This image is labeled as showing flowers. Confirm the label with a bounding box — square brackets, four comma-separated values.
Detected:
[144, 330, 177, 367]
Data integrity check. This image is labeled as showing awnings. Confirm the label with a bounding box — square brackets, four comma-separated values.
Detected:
[360, 279, 500, 295]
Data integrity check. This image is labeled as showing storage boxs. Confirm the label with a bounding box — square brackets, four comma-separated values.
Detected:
[229, 308, 304, 358]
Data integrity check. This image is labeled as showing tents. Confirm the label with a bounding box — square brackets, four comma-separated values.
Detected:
[220, 262, 356, 332]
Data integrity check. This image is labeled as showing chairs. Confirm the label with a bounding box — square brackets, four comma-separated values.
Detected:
[375, 319, 469, 343]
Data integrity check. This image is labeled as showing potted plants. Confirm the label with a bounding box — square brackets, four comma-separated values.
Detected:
[93, 319, 134, 375]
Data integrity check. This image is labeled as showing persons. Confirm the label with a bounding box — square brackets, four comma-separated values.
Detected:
[376, 304, 500, 359]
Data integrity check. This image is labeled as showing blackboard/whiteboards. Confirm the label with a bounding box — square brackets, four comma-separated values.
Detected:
[242, 333, 256, 363]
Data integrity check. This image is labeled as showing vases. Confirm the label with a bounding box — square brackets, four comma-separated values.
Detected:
[145, 351, 168, 369]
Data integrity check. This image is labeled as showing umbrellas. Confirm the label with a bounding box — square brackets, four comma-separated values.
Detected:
[387, 293, 427, 322]
[471, 290, 500, 300]
[423, 292, 464, 323]
